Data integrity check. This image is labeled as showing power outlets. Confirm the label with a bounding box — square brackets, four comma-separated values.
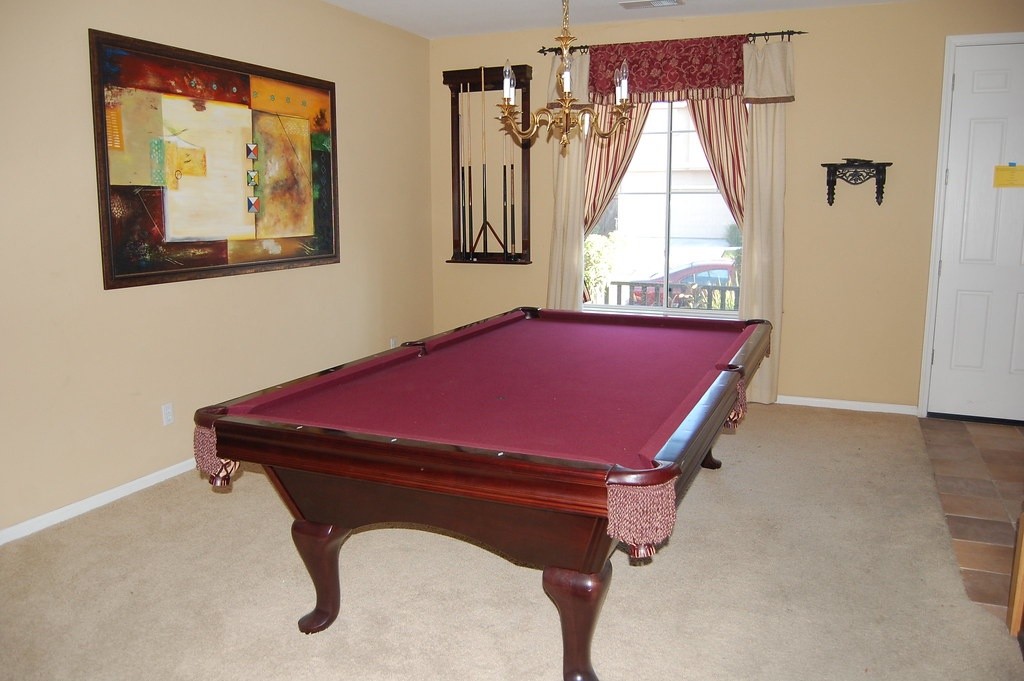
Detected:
[162, 403, 175, 425]
[391, 337, 398, 349]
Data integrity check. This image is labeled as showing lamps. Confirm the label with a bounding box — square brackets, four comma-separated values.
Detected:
[494, 0, 637, 148]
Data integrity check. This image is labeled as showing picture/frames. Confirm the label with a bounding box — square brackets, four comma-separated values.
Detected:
[88, 28, 340, 290]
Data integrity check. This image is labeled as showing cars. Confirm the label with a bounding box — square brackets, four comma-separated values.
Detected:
[632, 261, 738, 307]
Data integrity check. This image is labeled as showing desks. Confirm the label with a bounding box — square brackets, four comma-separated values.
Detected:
[195, 306, 773, 681]
